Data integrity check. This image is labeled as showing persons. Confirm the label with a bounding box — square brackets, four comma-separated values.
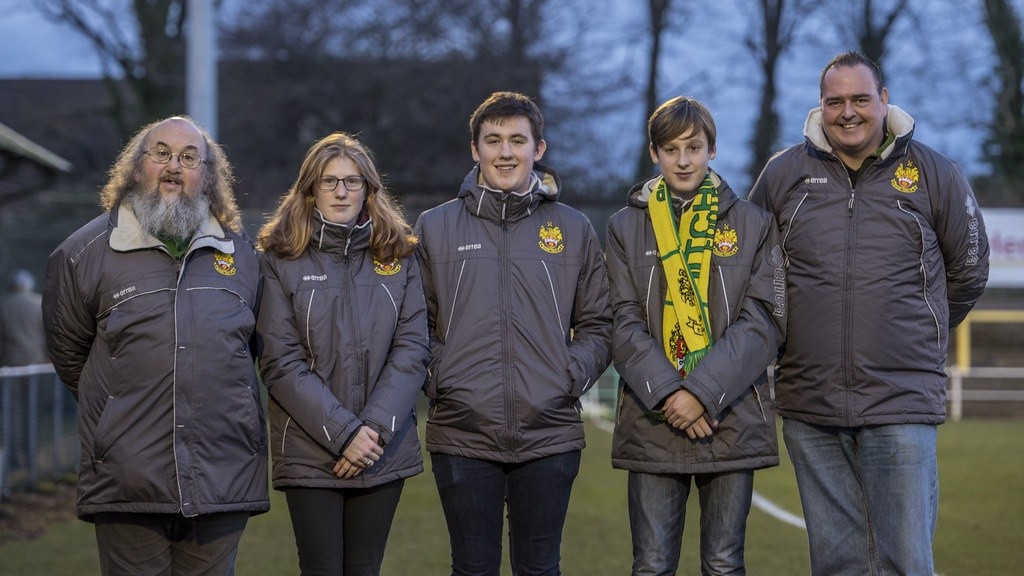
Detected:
[413, 93, 614, 576]
[254, 133, 430, 576]
[749, 49, 991, 576]
[606, 97, 791, 576]
[43, 114, 265, 576]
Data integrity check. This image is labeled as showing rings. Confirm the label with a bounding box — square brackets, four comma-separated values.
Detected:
[364, 459, 371, 466]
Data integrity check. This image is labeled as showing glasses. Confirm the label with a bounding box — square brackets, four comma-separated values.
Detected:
[317, 176, 366, 191]
[143, 147, 207, 169]
